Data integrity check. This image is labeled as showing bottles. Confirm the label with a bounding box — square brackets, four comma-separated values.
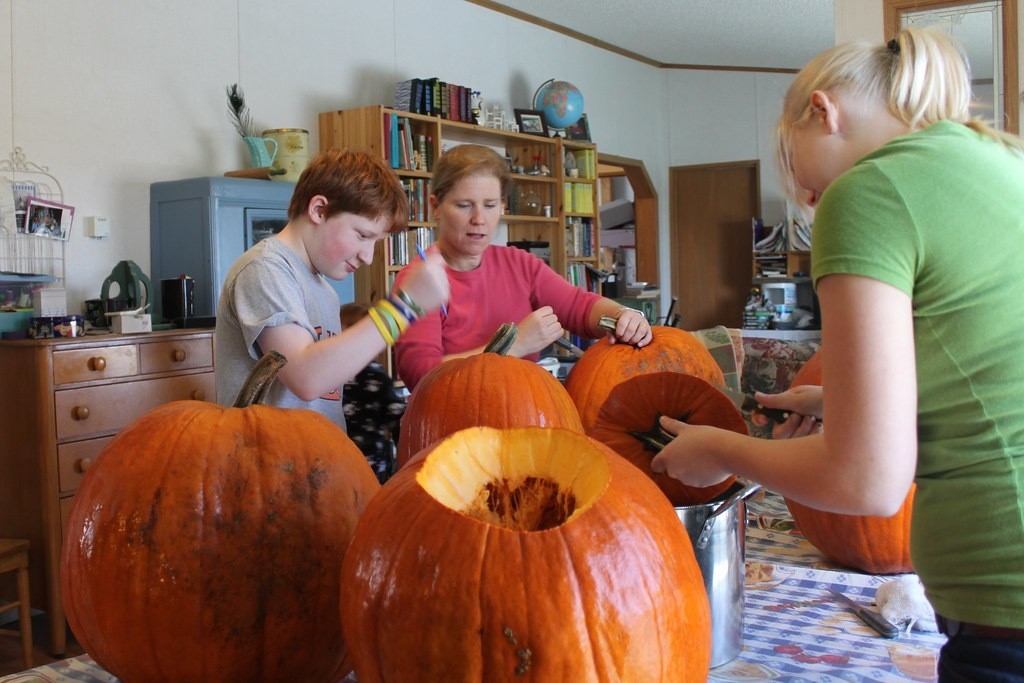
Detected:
[518, 190, 541, 215]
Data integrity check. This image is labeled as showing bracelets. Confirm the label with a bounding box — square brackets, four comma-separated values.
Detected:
[616, 307, 644, 316]
[368, 286, 426, 346]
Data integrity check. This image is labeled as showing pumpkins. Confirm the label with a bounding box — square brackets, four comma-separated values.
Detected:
[337, 427, 710, 683]
[783, 349, 918, 574]
[399, 322, 586, 470]
[58, 351, 381, 683]
[566, 314, 751, 508]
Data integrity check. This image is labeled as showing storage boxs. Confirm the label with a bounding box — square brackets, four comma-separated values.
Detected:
[599, 198, 637, 248]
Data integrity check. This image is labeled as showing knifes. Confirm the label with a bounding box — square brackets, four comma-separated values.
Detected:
[825, 587, 898, 637]
[711, 381, 791, 426]
[556, 338, 586, 361]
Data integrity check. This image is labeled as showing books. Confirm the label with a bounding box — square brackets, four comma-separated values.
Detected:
[567, 262, 596, 294]
[388, 227, 435, 265]
[566, 217, 595, 257]
[393, 78, 472, 123]
[384, 114, 433, 172]
[564, 182, 593, 213]
[389, 271, 397, 297]
[398, 178, 433, 222]
[573, 149, 595, 179]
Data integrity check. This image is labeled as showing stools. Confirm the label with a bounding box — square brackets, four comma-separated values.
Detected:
[1, 538, 35, 669]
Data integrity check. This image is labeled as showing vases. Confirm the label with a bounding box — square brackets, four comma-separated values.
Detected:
[244, 135, 279, 167]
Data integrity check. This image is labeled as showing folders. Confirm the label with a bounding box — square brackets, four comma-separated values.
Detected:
[384, 113, 391, 159]
[391, 114, 400, 169]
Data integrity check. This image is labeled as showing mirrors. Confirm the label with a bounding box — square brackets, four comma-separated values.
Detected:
[882, 0, 1020, 134]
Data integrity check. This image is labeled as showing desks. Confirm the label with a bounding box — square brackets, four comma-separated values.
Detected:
[703, 493, 948, 682]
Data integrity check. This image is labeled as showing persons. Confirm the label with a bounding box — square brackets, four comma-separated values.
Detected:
[649, 26, 1024, 683]
[214, 146, 449, 437]
[391, 144, 652, 394]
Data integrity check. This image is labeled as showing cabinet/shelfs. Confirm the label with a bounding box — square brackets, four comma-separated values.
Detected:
[0, 325, 216, 661]
[150, 178, 356, 330]
[317, 104, 601, 381]
[752, 217, 811, 283]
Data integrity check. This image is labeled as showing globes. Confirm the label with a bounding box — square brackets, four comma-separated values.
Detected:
[532, 78, 584, 138]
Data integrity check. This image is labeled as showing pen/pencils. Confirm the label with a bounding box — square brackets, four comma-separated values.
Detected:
[417, 244, 449, 318]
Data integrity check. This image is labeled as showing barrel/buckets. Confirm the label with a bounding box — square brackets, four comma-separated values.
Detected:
[663, 480, 762, 667]
[263, 128, 309, 181]
